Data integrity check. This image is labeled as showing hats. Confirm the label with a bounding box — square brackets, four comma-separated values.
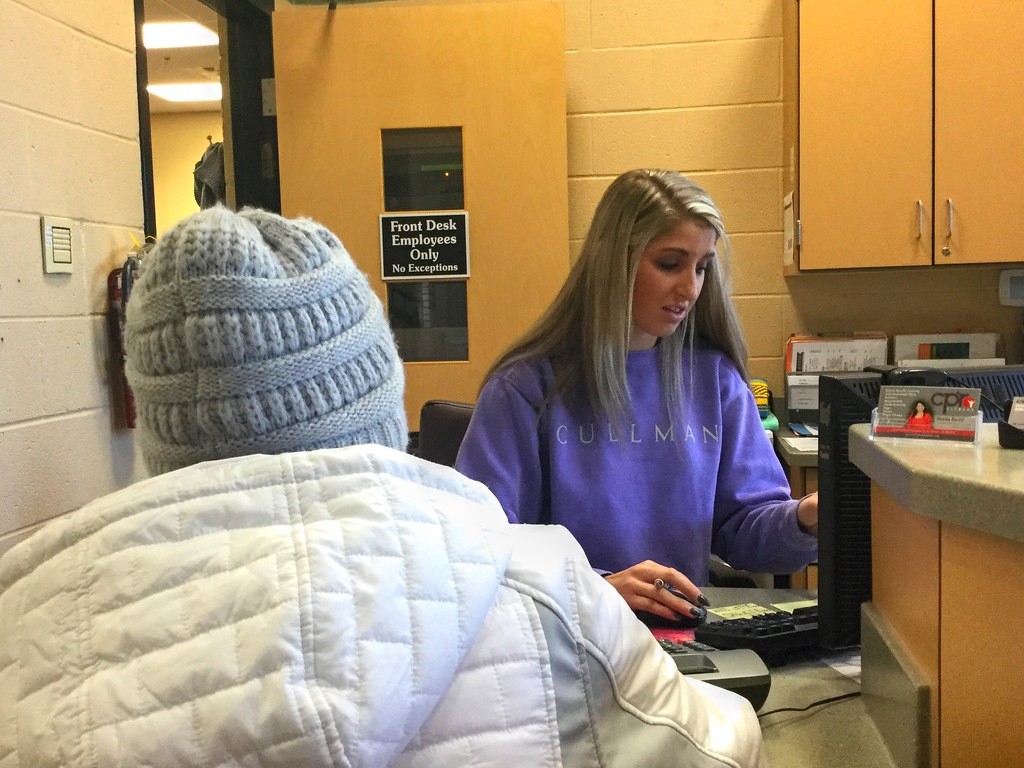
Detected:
[122, 202, 407, 482]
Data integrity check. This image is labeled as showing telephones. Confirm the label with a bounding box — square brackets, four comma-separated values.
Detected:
[655, 636, 772, 715]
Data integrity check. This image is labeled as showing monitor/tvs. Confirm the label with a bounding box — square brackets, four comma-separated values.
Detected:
[814, 363, 1024, 654]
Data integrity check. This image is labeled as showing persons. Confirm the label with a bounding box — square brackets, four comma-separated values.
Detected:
[454, 168, 817, 624]
[0, 203, 770, 768]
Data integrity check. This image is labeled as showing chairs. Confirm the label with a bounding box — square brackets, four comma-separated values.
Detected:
[418, 398, 477, 470]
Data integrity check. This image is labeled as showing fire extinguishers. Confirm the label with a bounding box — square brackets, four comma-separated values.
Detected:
[109, 239, 155, 430]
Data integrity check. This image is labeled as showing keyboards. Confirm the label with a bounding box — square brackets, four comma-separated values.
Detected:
[694, 606, 821, 665]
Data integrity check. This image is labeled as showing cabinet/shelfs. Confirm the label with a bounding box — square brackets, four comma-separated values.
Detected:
[781, 0, 1024, 276]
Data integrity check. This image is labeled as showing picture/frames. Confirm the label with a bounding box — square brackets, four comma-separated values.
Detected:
[377, 210, 471, 284]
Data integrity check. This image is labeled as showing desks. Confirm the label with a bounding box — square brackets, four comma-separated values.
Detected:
[653, 590, 899, 768]
